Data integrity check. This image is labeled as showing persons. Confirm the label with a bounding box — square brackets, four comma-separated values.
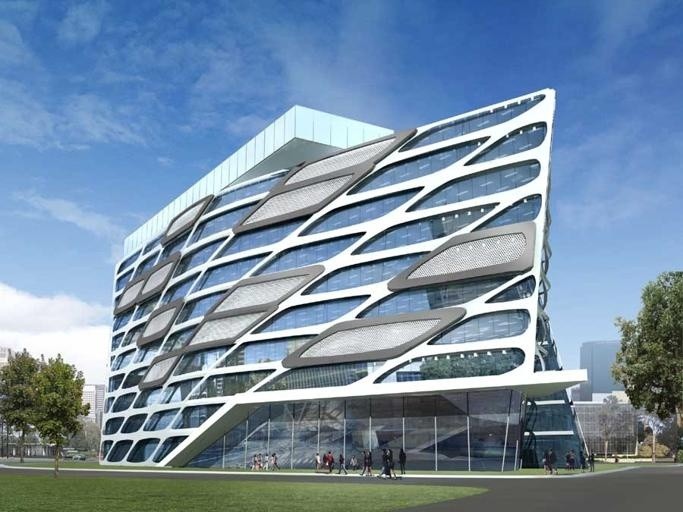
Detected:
[397, 448, 406, 474]
[538, 447, 595, 475]
[256, 453, 262, 471]
[268, 454, 278, 472]
[314, 448, 396, 480]
[249, 454, 258, 471]
[273, 452, 280, 470]
[261, 453, 268, 471]
